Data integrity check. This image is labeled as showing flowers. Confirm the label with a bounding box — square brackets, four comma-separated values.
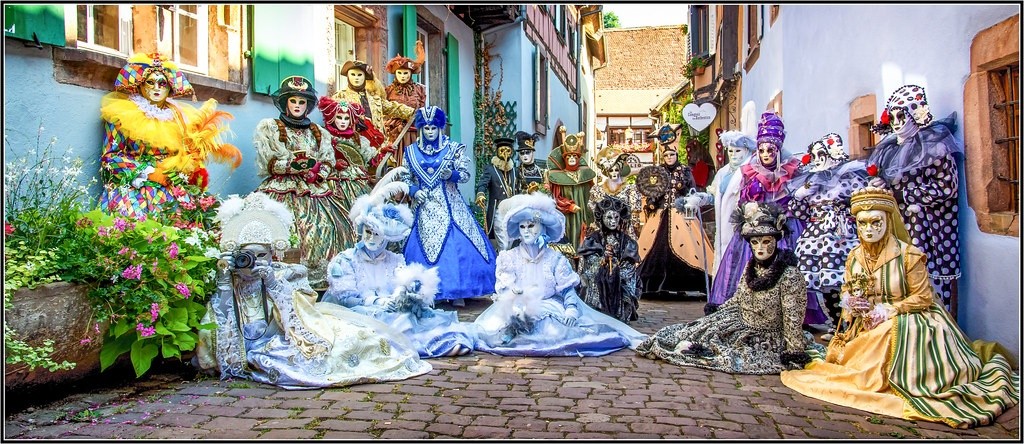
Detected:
[880, 109, 892, 127]
[799, 155, 810, 166]
[865, 161, 880, 182]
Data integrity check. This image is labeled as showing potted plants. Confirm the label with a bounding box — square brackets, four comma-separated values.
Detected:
[680, 54, 709, 80]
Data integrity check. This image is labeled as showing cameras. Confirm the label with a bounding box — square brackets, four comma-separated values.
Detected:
[232, 249, 255, 269]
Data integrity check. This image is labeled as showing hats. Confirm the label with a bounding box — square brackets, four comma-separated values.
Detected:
[341, 61, 374, 81]
[385, 41, 427, 74]
[756, 113, 785, 179]
[560, 132, 586, 158]
[648, 124, 683, 155]
[493, 191, 566, 250]
[719, 130, 757, 153]
[594, 196, 629, 232]
[807, 132, 847, 161]
[729, 201, 791, 242]
[596, 147, 630, 178]
[514, 131, 540, 151]
[870, 85, 932, 135]
[347, 167, 415, 242]
[115, 52, 198, 104]
[272, 76, 318, 117]
[217, 191, 295, 260]
[850, 166, 912, 244]
[494, 138, 514, 148]
[319, 97, 366, 129]
[414, 106, 445, 150]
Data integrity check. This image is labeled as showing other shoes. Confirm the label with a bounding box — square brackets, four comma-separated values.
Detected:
[677, 291, 688, 300]
[452, 299, 466, 307]
[821, 334, 834, 341]
[443, 344, 470, 356]
[659, 291, 673, 301]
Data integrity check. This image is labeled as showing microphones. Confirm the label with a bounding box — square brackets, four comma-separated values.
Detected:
[606, 244, 612, 277]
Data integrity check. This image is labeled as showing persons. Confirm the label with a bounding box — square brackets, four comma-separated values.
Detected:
[99, 57, 1020, 428]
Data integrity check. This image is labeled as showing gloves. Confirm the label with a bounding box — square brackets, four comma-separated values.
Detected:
[250, 261, 276, 287]
[780, 352, 810, 370]
[391, 286, 407, 309]
[528, 181, 538, 194]
[705, 304, 723, 319]
[850, 299, 871, 315]
[476, 195, 487, 211]
[684, 196, 702, 214]
[439, 167, 453, 180]
[413, 190, 428, 208]
[219, 251, 235, 280]
[374, 297, 397, 313]
[794, 177, 818, 202]
[861, 304, 890, 330]
[564, 308, 580, 327]
